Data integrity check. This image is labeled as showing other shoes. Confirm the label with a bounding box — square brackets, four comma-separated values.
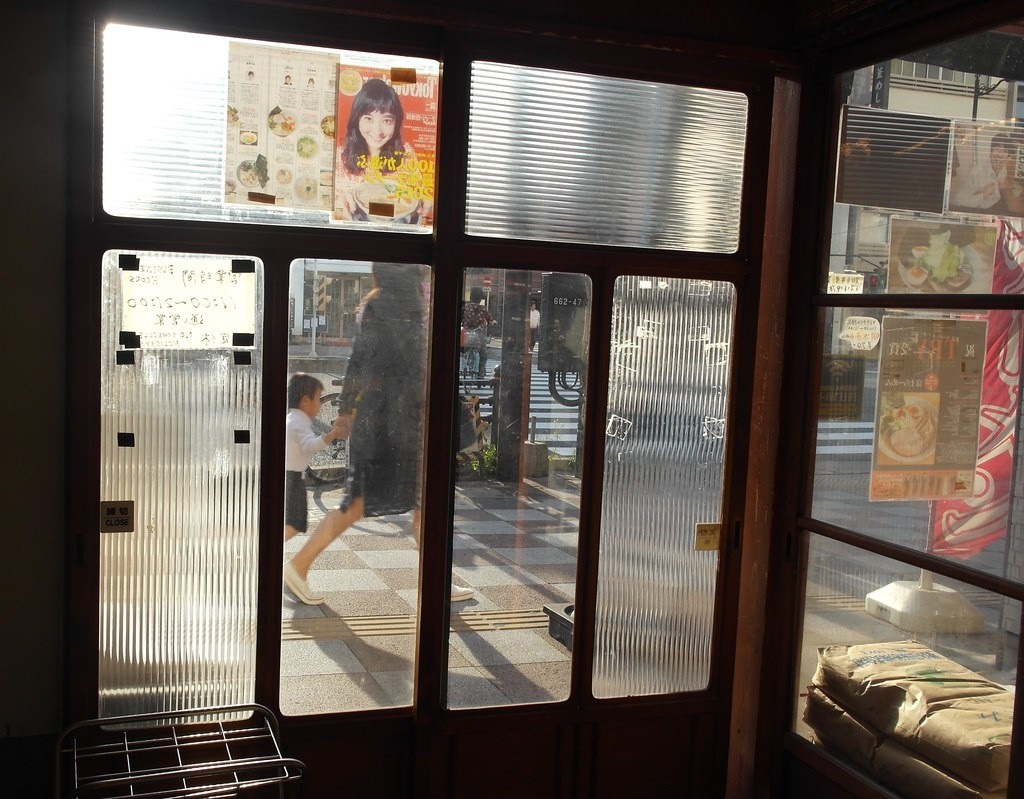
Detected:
[283, 560, 324, 604]
[451, 584, 474, 601]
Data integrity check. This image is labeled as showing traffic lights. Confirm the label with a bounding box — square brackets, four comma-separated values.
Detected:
[869, 274, 878, 294]
[878, 276, 886, 289]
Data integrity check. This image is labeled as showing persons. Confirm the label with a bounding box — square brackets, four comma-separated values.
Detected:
[246, 71, 256, 83]
[307, 79, 315, 88]
[952, 133, 1024, 216]
[334, 78, 432, 223]
[284, 263, 474, 606]
[284, 75, 292, 86]
[461, 286, 497, 389]
[530, 301, 540, 352]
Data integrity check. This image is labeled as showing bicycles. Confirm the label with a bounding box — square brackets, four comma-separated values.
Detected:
[306, 376, 351, 485]
[462, 322, 494, 392]
